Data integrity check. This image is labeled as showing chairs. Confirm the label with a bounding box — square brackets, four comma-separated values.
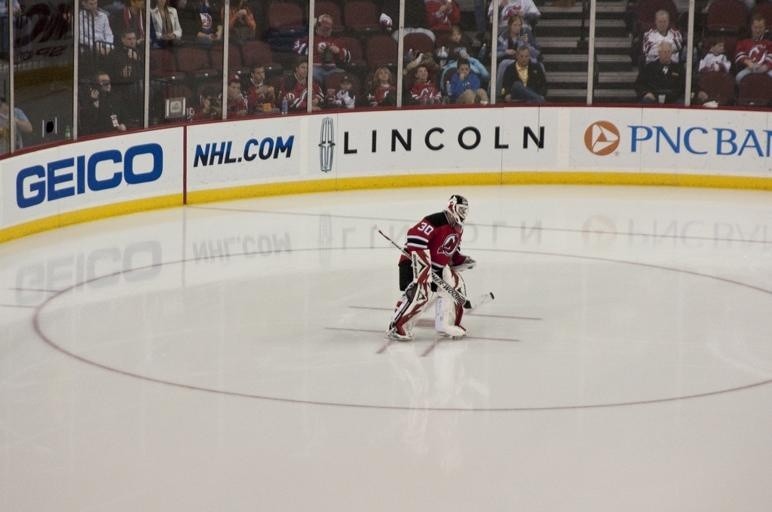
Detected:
[147, 1, 771, 108]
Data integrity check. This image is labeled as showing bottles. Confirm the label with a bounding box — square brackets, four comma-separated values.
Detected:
[446, 80, 452, 97]
[282, 95, 289, 114]
[64, 126, 72, 139]
[478, 43, 487, 62]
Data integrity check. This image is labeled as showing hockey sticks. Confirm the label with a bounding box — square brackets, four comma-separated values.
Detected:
[374, 225, 494, 308]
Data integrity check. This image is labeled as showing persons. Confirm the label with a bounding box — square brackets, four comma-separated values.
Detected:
[0, 0, 546, 158]
[385, 191, 474, 340]
[624, 0, 772, 111]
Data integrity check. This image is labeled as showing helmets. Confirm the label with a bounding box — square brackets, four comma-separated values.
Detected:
[445, 194, 469, 226]
